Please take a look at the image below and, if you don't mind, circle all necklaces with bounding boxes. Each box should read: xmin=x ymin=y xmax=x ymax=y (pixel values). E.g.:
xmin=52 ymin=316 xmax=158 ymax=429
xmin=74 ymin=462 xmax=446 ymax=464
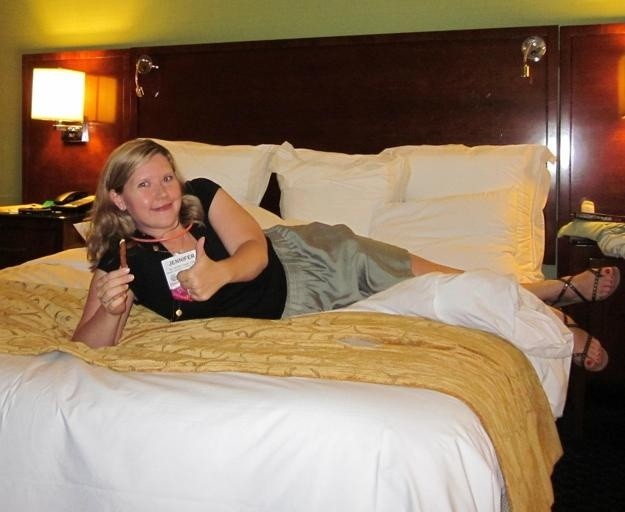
xmin=130 ymin=217 xmax=193 ymax=243
xmin=152 ymin=220 xmax=183 ymax=322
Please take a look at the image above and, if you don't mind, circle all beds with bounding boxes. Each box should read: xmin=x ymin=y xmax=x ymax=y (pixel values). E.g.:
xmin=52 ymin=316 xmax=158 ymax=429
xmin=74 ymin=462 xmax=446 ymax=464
xmin=0 ymin=217 xmax=575 ymax=510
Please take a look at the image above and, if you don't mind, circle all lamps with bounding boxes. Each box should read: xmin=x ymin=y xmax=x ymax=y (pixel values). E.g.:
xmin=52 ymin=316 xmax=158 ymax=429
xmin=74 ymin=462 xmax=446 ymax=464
xmin=520 ymin=34 xmax=548 ymax=77
xmin=133 ymin=57 xmax=160 ymax=99
xmin=30 ymin=66 xmax=90 ymax=144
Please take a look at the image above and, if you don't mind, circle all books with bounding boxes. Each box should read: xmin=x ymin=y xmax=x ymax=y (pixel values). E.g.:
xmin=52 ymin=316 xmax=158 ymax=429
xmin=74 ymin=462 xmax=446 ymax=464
xmin=573 ymin=211 xmax=625 ymax=223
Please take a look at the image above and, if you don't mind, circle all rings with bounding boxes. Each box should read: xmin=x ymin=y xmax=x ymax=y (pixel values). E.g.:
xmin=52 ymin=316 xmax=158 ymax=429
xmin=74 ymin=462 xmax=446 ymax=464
xmin=187 ymin=287 xmax=191 ymax=296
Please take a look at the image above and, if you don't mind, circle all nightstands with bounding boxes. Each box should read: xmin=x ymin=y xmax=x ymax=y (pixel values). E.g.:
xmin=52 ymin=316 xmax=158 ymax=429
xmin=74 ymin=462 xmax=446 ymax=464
xmin=1 ymin=202 xmax=90 ymax=268
xmin=556 ymin=216 xmax=625 ymax=303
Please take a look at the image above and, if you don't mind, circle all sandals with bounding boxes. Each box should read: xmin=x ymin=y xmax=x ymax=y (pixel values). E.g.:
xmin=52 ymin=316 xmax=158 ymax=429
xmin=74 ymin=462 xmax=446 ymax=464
xmin=555 ymin=307 xmax=609 ymax=372
xmin=546 ymin=267 xmax=620 ymax=306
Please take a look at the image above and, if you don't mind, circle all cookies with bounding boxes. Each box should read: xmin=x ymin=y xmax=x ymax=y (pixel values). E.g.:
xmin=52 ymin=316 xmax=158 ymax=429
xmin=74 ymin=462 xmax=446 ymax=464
xmin=119 ymin=239 xmax=127 ymax=268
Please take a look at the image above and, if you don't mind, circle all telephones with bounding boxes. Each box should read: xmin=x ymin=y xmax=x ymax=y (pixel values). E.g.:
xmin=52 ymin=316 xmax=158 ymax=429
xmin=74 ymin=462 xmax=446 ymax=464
xmin=50 ymin=190 xmax=96 ymax=211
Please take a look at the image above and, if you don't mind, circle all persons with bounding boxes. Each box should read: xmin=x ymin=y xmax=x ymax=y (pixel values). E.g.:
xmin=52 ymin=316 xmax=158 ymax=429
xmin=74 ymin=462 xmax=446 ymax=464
xmin=71 ymin=138 xmax=621 ymax=373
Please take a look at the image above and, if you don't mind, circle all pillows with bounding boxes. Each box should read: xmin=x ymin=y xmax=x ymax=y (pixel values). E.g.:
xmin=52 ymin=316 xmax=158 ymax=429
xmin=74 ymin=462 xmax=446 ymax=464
xmin=271 ymin=141 xmax=409 ymax=231
xmin=377 ymin=142 xmax=554 ymax=282
xmin=366 ymin=182 xmax=536 ymax=281
xmin=135 ymin=138 xmax=279 ymax=209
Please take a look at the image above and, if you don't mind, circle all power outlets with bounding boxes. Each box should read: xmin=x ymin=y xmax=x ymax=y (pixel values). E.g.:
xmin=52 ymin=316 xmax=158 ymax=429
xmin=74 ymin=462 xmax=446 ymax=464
xmin=581 ymin=202 xmax=594 ymax=213
